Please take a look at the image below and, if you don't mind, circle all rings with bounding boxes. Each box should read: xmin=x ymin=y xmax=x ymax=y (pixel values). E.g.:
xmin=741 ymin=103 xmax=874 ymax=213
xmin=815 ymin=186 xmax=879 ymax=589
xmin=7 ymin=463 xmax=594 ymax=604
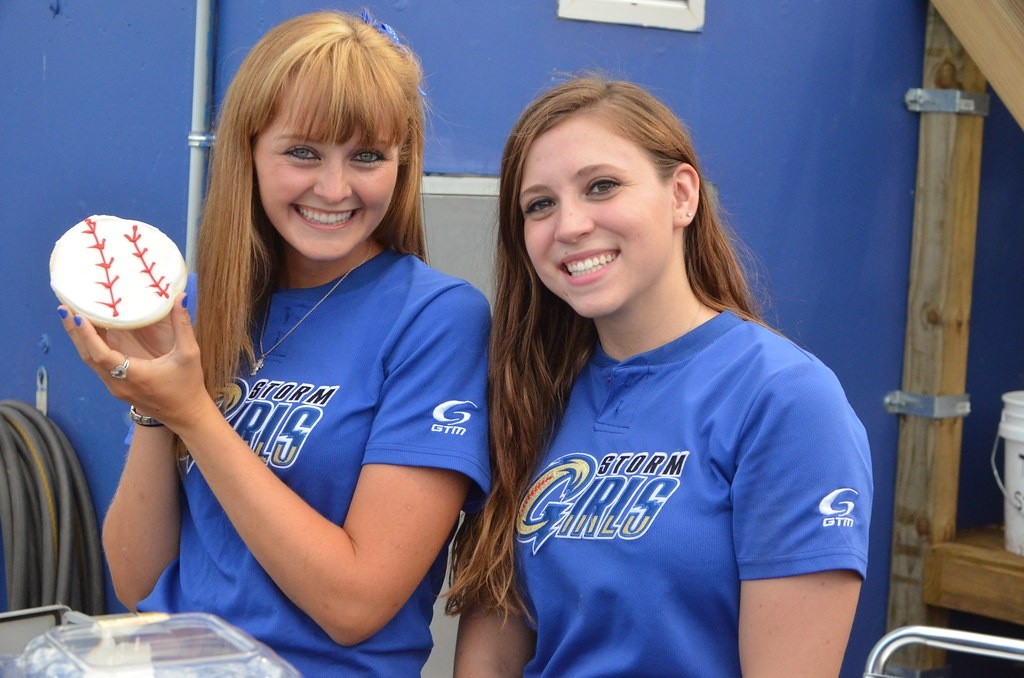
xmin=111 ymin=355 xmax=130 ymax=379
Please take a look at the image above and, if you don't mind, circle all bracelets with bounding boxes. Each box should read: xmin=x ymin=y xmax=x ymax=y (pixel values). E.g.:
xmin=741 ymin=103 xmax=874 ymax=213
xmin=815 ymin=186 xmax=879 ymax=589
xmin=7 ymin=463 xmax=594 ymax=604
xmin=130 ymin=405 xmax=163 ymax=427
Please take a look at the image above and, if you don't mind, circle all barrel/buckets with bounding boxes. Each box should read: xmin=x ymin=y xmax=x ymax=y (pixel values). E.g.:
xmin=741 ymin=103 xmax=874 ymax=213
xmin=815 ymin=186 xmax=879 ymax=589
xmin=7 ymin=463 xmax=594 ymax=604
xmin=990 ymin=390 xmax=1024 ymax=558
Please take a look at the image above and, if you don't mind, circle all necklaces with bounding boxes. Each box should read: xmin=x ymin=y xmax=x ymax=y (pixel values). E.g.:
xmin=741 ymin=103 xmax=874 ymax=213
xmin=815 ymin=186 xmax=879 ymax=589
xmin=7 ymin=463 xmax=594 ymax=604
xmin=250 ymin=241 xmax=373 ymax=376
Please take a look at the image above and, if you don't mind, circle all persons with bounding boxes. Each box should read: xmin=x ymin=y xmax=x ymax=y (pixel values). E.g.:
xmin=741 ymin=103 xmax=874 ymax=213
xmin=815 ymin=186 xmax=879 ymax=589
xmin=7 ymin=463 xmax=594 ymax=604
xmin=56 ymin=10 xmax=495 ymax=677
xmin=444 ymin=72 xmax=875 ymax=678
